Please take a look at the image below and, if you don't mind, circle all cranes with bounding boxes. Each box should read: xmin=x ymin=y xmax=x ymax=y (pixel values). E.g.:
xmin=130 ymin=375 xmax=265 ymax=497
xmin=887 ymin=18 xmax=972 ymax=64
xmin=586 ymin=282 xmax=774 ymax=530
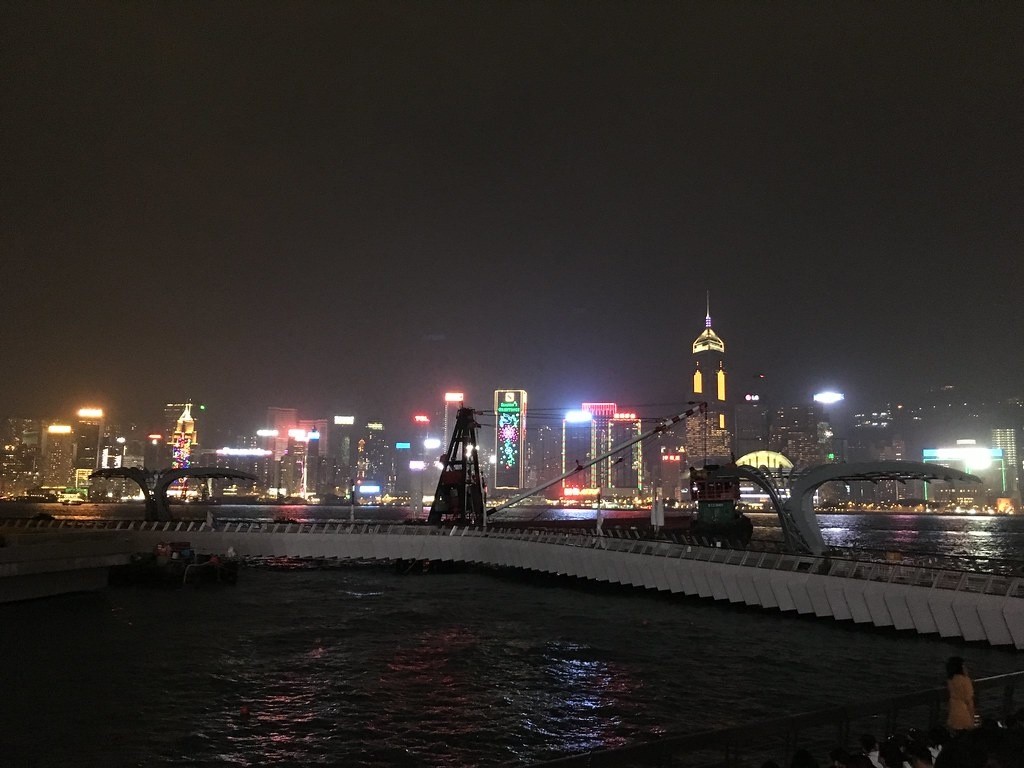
xmin=425 ymin=401 xmax=710 ymax=526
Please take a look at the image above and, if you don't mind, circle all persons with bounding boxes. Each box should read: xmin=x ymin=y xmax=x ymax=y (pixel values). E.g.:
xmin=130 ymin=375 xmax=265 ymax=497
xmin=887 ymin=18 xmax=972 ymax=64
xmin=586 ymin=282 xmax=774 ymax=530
xmin=759 ymin=681 xmax=1024 ymax=768
xmin=944 ymin=651 xmax=977 ymax=736
